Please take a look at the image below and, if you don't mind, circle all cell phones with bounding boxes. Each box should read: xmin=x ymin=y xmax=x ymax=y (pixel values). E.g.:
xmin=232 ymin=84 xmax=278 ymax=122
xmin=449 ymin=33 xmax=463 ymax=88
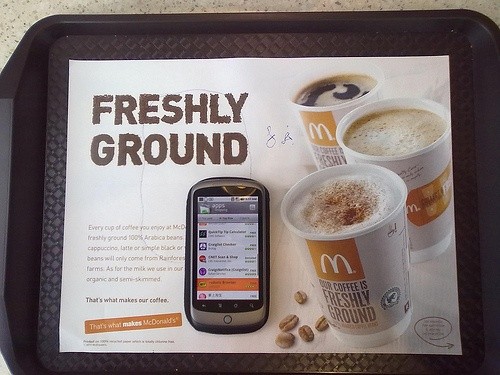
xmin=184 ymin=176 xmax=270 ymax=335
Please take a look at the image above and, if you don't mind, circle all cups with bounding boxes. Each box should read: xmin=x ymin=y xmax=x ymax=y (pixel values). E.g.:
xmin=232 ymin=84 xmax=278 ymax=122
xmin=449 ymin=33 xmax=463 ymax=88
xmin=336 ymin=95 xmax=455 ymax=262
xmin=289 ymin=74 xmax=384 ymax=171
xmin=281 ymin=163 xmax=412 ymax=348
